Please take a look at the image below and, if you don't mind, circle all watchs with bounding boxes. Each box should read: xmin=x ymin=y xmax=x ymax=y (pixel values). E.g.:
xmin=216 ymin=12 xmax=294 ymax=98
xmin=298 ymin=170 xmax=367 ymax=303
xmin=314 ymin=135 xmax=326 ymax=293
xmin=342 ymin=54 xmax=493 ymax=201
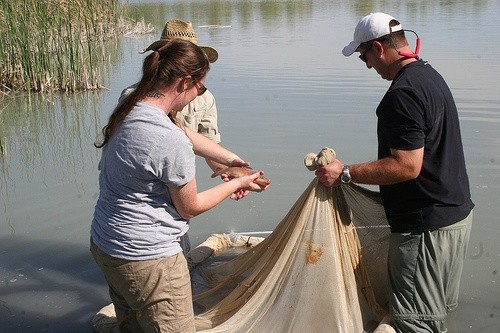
xmin=340 ymin=165 xmax=352 ymax=184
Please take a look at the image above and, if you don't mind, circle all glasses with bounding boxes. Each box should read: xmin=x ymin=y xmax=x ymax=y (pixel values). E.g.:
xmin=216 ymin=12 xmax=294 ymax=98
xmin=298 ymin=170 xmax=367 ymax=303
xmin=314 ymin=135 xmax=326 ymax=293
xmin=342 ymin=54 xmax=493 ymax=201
xmin=190 ymin=74 xmax=208 ymax=96
xmin=359 ymin=40 xmax=387 ymax=63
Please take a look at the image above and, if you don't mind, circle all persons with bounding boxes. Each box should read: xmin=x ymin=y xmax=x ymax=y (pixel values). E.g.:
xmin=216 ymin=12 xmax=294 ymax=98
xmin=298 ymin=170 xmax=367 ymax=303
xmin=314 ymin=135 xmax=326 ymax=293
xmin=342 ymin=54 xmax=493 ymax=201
xmin=89 ymin=18 xmax=269 ymax=333
xmin=315 ymin=12 xmax=475 ymax=333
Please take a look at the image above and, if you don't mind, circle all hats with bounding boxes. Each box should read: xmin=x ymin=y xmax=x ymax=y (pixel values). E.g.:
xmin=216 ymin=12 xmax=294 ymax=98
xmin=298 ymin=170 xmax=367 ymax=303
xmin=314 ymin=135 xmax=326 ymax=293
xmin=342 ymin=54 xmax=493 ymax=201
xmin=341 ymin=13 xmax=402 ymax=56
xmin=139 ymin=19 xmax=218 ymax=62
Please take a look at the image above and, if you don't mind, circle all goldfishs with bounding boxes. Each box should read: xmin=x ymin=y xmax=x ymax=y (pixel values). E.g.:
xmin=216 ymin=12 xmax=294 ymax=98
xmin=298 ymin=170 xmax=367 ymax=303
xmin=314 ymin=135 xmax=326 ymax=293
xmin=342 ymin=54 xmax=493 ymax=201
xmin=210 ymin=166 xmax=270 ymax=186
